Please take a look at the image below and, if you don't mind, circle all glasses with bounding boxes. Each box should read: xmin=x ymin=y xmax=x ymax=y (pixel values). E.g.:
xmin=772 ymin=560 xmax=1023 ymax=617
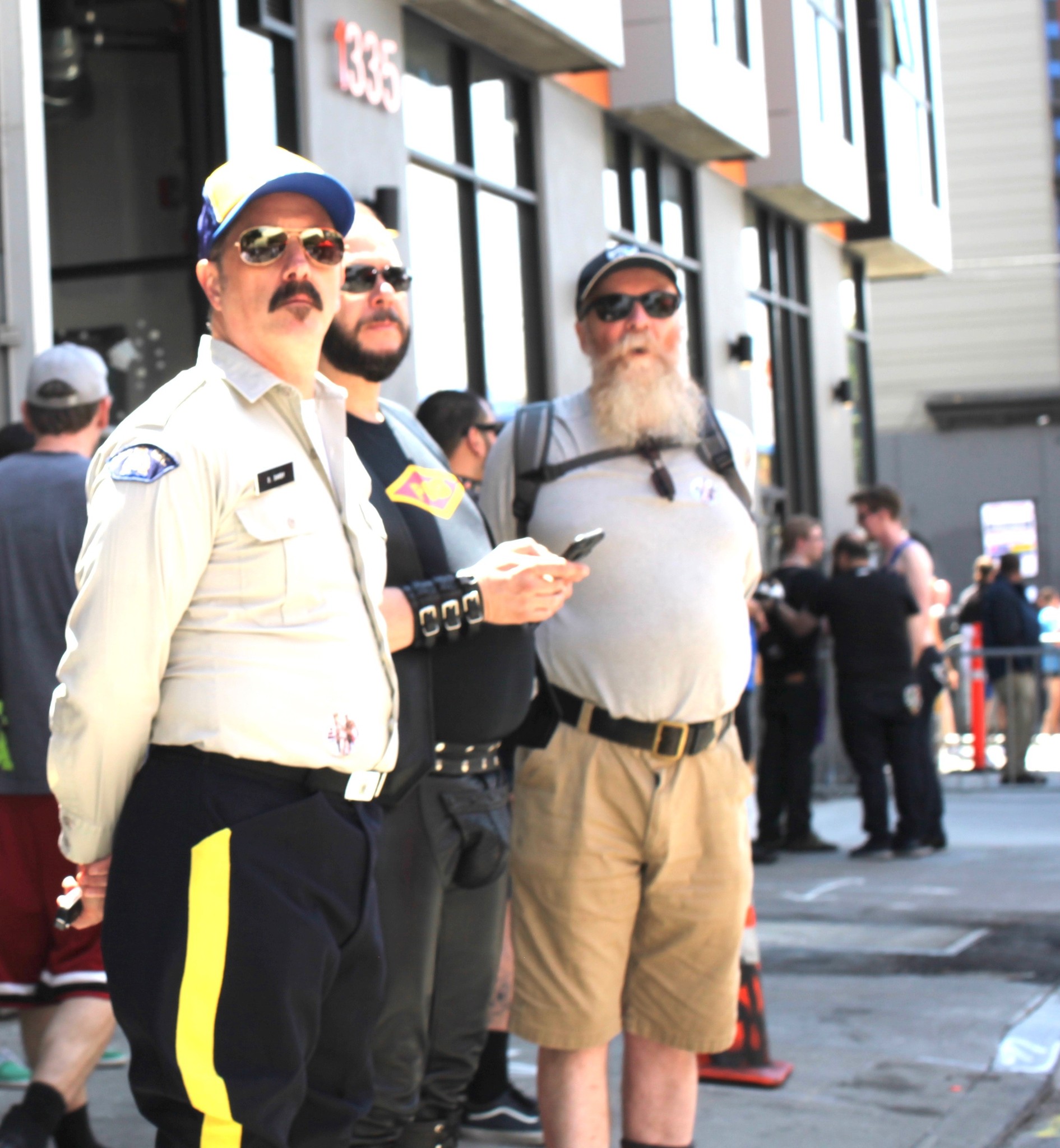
xmin=227 ymin=223 xmax=347 ymax=270
xmin=579 ymin=289 xmax=681 ymax=323
xmin=462 ymin=420 xmax=505 ymax=435
xmin=337 ymin=264 xmax=412 ymax=298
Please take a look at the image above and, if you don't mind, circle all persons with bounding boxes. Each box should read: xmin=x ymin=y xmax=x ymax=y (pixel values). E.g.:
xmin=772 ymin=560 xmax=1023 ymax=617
xmin=477 ymin=242 xmax=764 ymax=1147
xmin=0 ymin=144 xmax=1060 ymax=1148
xmin=47 ymin=139 xmax=403 ymax=1148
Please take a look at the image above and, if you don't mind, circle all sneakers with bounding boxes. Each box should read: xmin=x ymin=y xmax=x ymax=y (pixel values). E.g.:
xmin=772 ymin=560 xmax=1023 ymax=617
xmin=460 ymin=1082 xmax=546 ymax=1145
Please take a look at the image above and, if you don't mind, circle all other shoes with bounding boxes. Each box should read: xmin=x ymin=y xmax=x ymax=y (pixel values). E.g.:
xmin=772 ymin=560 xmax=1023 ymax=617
xmin=759 ymin=830 xmax=834 ymax=852
xmin=1002 ymin=773 xmax=1047 ymax=784
xmin=853 ymin=833 xmax=935 ymax=859
xmin=0 ymin=1104 xmax=49 ymax=1148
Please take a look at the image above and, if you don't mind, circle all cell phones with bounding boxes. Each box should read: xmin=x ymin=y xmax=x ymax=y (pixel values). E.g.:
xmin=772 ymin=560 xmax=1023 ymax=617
xmin=562 ymin=529 xmax=605 ymax=561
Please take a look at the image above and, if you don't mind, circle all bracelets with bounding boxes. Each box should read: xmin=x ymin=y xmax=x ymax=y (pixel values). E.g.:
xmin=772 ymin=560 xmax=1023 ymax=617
xmin=404 ymin=572 xmax=486 ymax=653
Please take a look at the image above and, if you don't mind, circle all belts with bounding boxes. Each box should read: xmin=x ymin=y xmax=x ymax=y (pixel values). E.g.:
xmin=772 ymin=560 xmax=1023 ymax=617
xmin=532 ymin=681 xmax=733 ymax=761
xmin=427 ymin=739 xmax=504 ymax=775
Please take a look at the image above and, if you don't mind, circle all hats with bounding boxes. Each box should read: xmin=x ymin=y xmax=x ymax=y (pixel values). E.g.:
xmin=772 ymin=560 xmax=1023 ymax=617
xmin=852 ymin=485 xmax=900 ymax=510
xmin=196 ymin=146 xmax=355 ymax=262
xmin=26 ymin=341 xmax=111 ymax=410
xmin=576 ymin=242 xmax=678 ymax=322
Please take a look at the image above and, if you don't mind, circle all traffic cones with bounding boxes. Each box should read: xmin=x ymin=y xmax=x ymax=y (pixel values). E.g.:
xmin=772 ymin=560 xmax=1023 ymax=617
xmin=698 ymin=904 xmax=796 ymax=1087
